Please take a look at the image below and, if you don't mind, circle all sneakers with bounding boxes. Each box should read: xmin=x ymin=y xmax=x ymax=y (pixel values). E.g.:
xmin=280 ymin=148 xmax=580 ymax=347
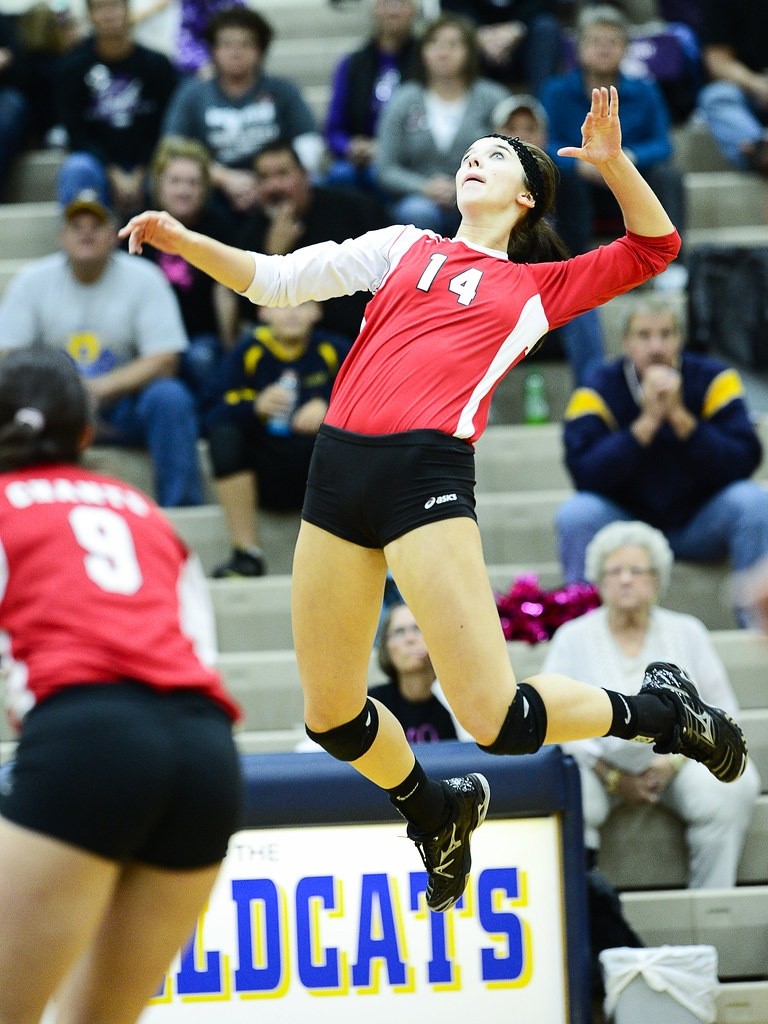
xmin=405 ymin=772 xmax=491 ymax=915
xmin=637 ymin=663 xmax=748 ymax=784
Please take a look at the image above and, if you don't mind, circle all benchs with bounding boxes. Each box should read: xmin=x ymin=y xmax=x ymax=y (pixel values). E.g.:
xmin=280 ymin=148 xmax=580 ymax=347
xmin=0 ymin=0 xmax=768 ymax=1024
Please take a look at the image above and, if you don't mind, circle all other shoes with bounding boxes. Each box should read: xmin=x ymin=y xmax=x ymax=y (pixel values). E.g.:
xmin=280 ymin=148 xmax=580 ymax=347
xmin=208 ymin=546 xmax=264 ymax=580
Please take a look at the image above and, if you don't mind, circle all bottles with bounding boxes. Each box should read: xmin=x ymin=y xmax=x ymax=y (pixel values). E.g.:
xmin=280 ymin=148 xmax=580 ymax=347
xmin=523 ymin=364 xmax=551 ymax=424
xmin=267 ymin=368 xmax=299 ymax=438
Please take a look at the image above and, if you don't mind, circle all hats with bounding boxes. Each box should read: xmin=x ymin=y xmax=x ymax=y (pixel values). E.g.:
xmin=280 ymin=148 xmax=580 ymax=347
xmin=63 ymin=184 xmax=123 ymax=230
xmin=493 ymin=93 xmax=550 ymax=133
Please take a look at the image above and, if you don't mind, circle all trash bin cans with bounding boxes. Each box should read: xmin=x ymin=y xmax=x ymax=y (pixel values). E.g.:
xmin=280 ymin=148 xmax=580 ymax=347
xmin=601 ymin=943 xmax=717 ymax=1024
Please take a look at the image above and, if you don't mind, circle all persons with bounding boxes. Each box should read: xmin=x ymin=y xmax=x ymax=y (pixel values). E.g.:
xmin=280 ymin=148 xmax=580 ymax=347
xmin=362 ymin=604 xmax=473 ymax=744
xmin=550 ymin=297 xmax=768 ymax=631
xmin=118 ymin=85 xmax=749 ymax=915
xmin=0 ymin=344 xmax=251 ymax=1024
xmin=532 ymin=519 xmax=763 ymax=891
xmin=0 ymin=0 xmax=345 ymax=577
xmin=324 ymin=0 xmax=768 ymax=393
